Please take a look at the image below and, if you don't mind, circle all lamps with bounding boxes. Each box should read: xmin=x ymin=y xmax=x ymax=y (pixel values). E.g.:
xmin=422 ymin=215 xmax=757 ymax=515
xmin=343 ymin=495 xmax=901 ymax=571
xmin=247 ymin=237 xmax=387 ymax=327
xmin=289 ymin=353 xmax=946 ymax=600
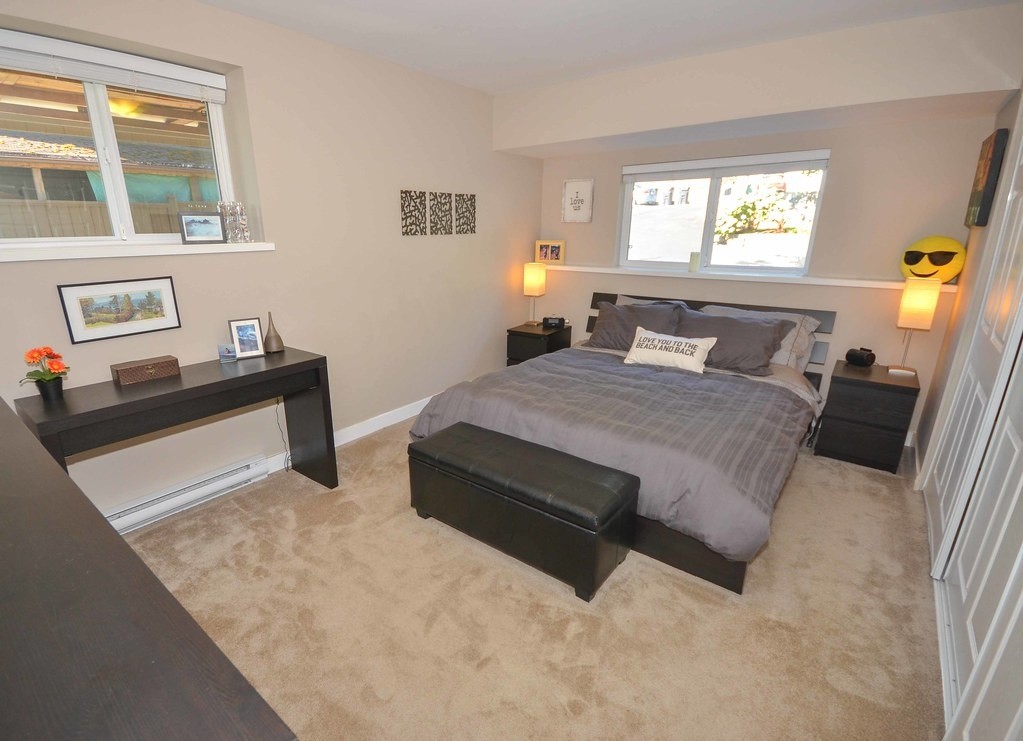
xmin=888 ymin=278 xmax=943 ymax=375
xmin=523 ymin=263 xmax=545 ymax=326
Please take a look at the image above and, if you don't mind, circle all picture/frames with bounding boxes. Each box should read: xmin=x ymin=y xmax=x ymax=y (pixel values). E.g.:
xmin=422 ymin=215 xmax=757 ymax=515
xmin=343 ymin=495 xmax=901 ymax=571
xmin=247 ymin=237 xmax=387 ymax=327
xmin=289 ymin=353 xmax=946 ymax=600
xmin=536 ymin=239 xmax=565 ymax=264
xmin=56 ymin=276 xmax=182 ymax=345
xmin=180 ymin=214 xmax=226 ymax=244
xmin=562 ymin=177 xmax=595 ymax=222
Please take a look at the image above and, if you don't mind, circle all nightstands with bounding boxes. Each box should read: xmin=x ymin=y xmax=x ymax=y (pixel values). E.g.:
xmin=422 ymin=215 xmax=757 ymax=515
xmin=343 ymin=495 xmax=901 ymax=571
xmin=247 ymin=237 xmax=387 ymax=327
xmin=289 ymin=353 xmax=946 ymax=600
xmin=507 ymin=321 xmax=571 ymax=367
xmin=813 ymin=360 xmax=920 ymax=474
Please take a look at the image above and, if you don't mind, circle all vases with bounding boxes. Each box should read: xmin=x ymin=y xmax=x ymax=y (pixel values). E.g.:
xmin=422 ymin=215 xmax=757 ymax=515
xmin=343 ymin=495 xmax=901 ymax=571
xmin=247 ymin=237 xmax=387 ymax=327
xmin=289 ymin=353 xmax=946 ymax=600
xmin=34 ymin=377 xmax=64 ymax=400
xmin=264 ymin=311 xmax=284 ymax=352
xmin=228 ymin=318 xmax=266 ymax=360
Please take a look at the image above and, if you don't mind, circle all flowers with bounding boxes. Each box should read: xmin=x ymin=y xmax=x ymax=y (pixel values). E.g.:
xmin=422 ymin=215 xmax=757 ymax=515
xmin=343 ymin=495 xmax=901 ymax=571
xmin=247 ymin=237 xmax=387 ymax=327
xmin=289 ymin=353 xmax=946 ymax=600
xmin=19 ymin=346 xmax=70 ymax=383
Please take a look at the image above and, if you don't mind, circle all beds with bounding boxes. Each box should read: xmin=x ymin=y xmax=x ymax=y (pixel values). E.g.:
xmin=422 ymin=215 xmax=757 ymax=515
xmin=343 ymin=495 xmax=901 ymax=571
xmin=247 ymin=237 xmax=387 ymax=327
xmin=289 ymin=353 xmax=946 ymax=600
xmin=408 ymin=289 xmax=837 ymax=597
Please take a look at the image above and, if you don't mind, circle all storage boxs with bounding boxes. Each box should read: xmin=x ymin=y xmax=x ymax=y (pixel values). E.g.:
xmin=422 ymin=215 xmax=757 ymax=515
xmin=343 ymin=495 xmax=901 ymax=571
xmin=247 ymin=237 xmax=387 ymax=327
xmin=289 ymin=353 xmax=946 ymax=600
xmin=110 ymin=355 xmax=181 ymax=386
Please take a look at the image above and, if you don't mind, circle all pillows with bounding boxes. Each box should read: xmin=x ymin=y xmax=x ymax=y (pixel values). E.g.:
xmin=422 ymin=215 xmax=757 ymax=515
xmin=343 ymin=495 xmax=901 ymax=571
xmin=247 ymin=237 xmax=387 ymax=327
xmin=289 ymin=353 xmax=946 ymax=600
xmin=617 ymin=293 xmax=686 ymax=305
xmin=624 ymin=325 xmax=717 ymax=373
xmin=580 ymin=301 xmax=675 ymax=350
xmin=674 ymin=304 xmax=797 ymax=375
xmin=699 ymin=304 xmax=820 ymax=369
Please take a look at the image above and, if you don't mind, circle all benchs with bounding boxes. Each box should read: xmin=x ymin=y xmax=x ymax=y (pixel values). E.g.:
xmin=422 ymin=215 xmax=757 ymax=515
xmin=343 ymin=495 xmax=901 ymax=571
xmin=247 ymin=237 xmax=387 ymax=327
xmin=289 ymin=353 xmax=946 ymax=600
xmin=408 ymin=420 xmax=640 ymax=603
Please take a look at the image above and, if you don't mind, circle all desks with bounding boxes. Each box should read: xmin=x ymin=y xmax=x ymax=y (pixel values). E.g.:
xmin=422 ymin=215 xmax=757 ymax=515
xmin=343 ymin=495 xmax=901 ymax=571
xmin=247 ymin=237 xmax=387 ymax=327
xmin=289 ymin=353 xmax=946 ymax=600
xmin=14 ymin=345 xmax=339 ymax=490
